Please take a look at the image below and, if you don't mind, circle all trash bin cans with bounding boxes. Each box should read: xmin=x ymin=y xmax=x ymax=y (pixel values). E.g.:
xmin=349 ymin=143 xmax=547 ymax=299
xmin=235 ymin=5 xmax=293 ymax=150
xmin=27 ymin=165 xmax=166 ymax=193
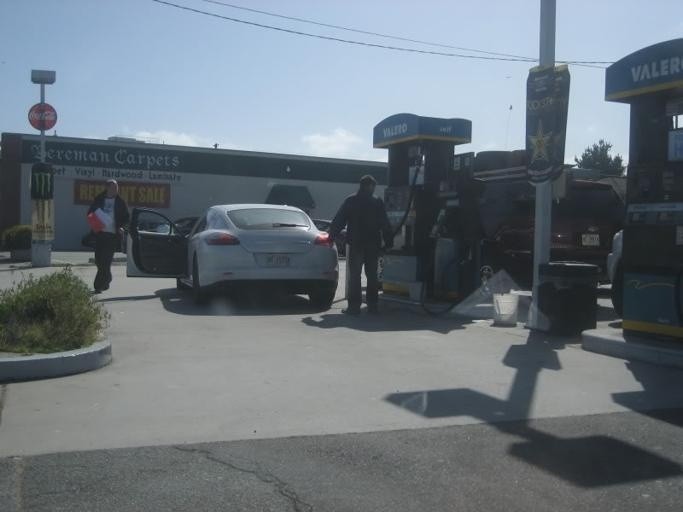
xmin=537 ymin=260 xmax=599 ymax=337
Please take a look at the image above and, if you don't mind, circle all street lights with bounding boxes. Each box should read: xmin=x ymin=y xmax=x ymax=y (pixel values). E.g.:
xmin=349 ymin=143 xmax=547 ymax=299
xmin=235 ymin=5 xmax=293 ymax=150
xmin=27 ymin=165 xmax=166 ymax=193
xmin=28 ymin=68 xmax=57 ymax=270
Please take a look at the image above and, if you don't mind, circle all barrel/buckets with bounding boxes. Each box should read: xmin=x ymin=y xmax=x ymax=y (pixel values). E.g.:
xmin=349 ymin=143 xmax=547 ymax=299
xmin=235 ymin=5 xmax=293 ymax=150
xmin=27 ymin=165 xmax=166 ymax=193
xmin=493 ymin=293 xmax=520 ymax=327
xmin=408 ymin=281 xmax=423 ymax=302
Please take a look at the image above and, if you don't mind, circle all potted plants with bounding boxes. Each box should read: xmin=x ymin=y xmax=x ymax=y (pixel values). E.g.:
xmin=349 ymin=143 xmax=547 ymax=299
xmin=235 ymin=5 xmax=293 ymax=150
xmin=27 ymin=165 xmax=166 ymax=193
xmin=3 ymin=223 xmax=31 ymax=262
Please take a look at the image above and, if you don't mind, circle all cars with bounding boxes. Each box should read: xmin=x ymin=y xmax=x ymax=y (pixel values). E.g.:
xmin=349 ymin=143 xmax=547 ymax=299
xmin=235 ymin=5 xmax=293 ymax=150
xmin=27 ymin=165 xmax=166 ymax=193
xmin=79 ymin=215 xmax=203 ymax=256
xmin=309 ymin=216 xmax=349 ymax=256
xmin=124 ymin=203 xmax=338 ymax=310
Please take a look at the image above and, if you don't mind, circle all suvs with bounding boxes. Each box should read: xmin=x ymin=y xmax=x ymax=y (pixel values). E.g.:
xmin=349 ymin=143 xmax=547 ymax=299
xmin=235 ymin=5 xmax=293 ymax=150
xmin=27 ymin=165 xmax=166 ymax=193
xmin=362 ymin=165 xmax=627 ymax=304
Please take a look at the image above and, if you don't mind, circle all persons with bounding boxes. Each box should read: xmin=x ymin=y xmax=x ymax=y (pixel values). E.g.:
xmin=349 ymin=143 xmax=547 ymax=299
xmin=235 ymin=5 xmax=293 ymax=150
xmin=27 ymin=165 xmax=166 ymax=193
xmin=327 ymin=176 xmax=392 ymax=315
xmin=87 ymin=179 xmax=130 ymax=293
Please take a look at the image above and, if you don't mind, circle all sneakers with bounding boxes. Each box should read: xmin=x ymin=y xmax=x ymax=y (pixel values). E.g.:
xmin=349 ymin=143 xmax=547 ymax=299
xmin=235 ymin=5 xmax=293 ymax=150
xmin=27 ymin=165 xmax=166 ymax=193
xmin=94 ymin=278 xmax=112 ymax=294
xmin=342 ymin=305 xmax=377 ymax=315
